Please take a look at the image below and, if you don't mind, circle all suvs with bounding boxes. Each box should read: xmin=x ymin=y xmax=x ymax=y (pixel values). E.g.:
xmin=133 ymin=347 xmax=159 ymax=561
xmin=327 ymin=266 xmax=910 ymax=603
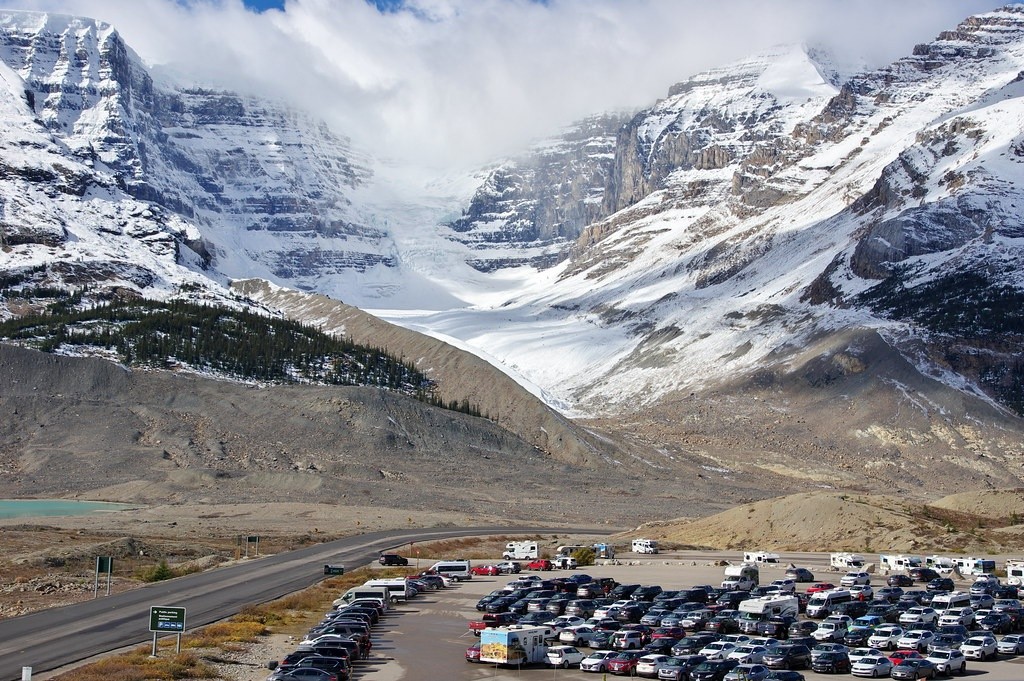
xmin=265 ymin=559 xmax=473 ymax=681
xmin=379 ymin=555 xmax=409 ymax=566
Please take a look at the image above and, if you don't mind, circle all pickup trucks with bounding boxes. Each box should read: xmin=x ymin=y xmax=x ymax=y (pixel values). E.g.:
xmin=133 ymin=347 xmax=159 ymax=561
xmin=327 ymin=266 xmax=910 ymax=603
xmin=468 ymin=613 xmax=517 ymax=637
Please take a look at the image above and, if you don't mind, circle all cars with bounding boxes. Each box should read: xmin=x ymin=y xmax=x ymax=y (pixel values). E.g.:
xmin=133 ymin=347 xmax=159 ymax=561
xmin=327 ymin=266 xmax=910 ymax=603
xmin=476 ymin=550 xmax=1024 ymax=681
xmin=465 ymin=640 xmax=482 ymax=662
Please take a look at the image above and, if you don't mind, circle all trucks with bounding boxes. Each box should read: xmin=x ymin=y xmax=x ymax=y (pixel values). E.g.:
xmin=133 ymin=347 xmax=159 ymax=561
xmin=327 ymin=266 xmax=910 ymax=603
xmin=555 ymin=544 xmax=593 ymax=559
xmin=502 ymin=539 xmax=540 ymax=560
xmin=632 ymin=539 xmax=659 ymax=554
xmin=594 ymin=543 xmax=616 ymax=559
